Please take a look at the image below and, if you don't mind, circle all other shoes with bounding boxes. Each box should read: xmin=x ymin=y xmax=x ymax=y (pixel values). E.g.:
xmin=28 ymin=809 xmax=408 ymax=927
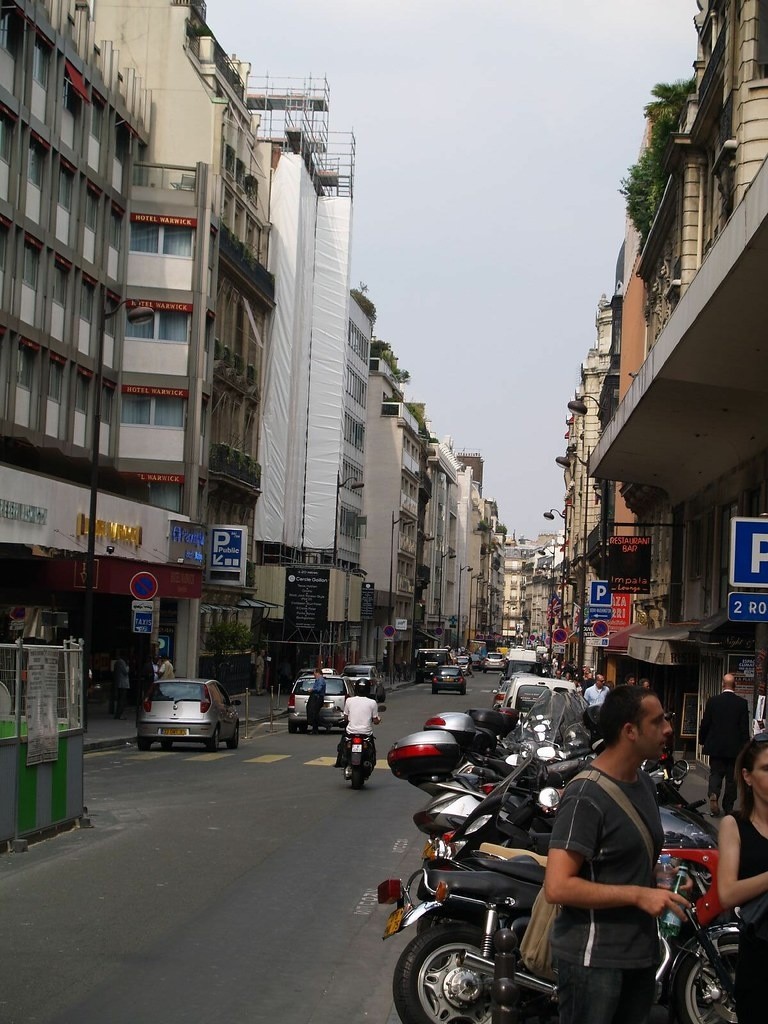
xmin=309 ymin=729 xmax=317 ymax=734
xmin=326 ymin=722 xmax=333 ymax=734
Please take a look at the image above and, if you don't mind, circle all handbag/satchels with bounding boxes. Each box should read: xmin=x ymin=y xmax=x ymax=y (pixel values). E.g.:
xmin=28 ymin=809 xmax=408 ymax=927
xmin=519 ymin=881 xmax=563 ymax=978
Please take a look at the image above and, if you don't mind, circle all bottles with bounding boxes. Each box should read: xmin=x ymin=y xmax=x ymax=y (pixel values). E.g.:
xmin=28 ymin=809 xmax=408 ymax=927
xmin=660 ymin=862 xmax=688 ymax=936
xmin=657 ymin=855 xmax=674 ymax=890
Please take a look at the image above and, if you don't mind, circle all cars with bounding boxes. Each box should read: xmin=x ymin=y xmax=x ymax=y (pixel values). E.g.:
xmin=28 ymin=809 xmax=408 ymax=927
xmin=481 ymin=651 xmax=508 ymax=674
xmin=430 ymin=664 xmax=469 ymax=695
xmin=134 ymin=678 xmax=242 ymax=752
xmin=287 ymin=673 xmax=356 ymax=734
xmin=341 ymin=661 xmax=386 ymax=703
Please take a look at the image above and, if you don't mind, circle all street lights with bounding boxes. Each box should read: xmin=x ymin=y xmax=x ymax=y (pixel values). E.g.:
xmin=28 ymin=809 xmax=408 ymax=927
xmin=384 ymin=510 xmax=413 ymax=676
xmin=537 ymin=544 xmax=558 ymax=677
xmin=76 ymin=286 xmax=154 ymax=735
xmin=467 ymin=571 xmax=502 ymax=642
xmin=436 ymin=540 xmax=458 ymax=649
xmin=455 ymin=563 xmax=474 ymax=651
xmin=567 ymin=383 xmax=612 ymax=682
xmin=555 ymin=443 xmax=591 ymax=684
xmin=543 ymin=503 xmax=568 ymax=676
xmin=332 ymin=469 xmax=365 ymax=566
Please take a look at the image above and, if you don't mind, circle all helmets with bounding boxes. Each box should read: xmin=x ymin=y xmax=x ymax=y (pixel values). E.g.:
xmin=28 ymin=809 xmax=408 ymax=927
xmin=354 ymin=679 xmax=371 ymax=696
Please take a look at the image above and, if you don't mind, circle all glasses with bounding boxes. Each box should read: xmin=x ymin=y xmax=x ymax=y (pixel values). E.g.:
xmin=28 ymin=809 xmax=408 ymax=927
xmin=597 ymin=679 xmax=604 ymax=683
xmin=752 ymin=733 xmax=768 ymax=741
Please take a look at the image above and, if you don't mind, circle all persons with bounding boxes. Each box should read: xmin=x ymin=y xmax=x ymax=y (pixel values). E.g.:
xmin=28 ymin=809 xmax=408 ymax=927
xmin=698 ymin=673 xmax=749 ymax=816
xmin=639 ymin=679 xmax=650 ymax=688
xmin=396 ymin=657 xmax=407 ymax=680
xmin=144 ymin=654 xmax=174 ymax=681
xmin=585 ymin=674 xmax=609 ymax=707
xmin=308 ymin=668 xmax=333 ymax=735
xmin=624 ymin=673 xmax=636 ymax=685
xmin=255 ymin=650 xmax=266 ymax=695
xmin=114 ymin=651 xmax=130 ymax=720
xmin=555 ymin=658 xmax=596 ymax=696
xmin=717 ymin=732 xmax=768 ymax=1024
xmin=544 ymin=685 xmax=694 ymax=1024
xmin=278 ymin=656 xmax=292 ymax=692
xmin=335 ymin=678 xmax=381 ymax=767
xmin=466 ymin=653 xmax=475 ymax=678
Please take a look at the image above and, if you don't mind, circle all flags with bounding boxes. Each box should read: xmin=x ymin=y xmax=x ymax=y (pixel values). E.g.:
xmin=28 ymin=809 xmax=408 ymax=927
xmin=547 ymin=593 xmax=561 ymax=625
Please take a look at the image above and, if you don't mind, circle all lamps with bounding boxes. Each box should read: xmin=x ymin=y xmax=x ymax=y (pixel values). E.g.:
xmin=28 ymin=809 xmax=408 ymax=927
xmin=106 ymin=545 xmax=115 ymax=555
xmin=177 ymin=557 xmax=184 ymax=563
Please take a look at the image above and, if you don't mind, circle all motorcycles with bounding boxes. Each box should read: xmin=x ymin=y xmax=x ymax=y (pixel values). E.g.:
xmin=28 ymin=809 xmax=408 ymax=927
xmin=332 ymin=705 xmax=387 ymax=789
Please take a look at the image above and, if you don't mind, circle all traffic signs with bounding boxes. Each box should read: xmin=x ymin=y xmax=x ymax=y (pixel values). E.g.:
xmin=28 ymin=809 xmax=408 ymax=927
xmin=588 ymin=579 xmax=612 ymax=620
xmin=204 ymin=523 xmax=249 ymax=586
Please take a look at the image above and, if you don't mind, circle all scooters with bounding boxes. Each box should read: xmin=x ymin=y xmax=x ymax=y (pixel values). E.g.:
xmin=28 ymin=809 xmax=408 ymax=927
xmin=375 ymin=643 xmax=745 ymax=1024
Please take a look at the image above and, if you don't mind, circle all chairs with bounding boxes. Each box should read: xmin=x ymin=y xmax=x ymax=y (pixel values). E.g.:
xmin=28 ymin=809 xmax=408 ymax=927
xmin=170 ymin=173 xmax=196 ymax=190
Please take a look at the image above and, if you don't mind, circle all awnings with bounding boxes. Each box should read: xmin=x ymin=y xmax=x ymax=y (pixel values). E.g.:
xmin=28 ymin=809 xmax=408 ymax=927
xmin=627 ymin=625 xmax=697 ymax=665
xmin=604 ymin=622 xmax=642 ymax=656
xmin=688 ymin=607 xmax=755 ymax=651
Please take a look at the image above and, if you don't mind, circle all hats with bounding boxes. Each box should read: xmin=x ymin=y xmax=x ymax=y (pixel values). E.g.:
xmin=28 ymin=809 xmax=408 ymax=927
xmin=158 ymin=652 xmax=170 ymax=659
